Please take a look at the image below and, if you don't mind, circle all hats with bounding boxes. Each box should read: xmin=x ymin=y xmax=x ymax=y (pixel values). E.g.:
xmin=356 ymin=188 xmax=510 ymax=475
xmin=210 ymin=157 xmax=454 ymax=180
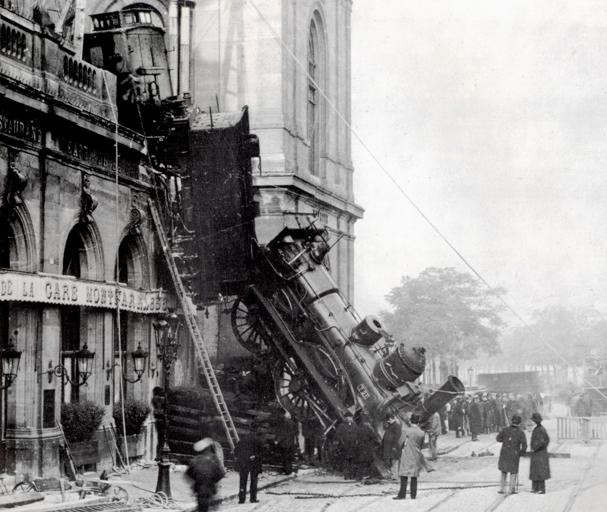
xmin=511 ymin=415 xmax=521 ymax=423
xmin=411 ymin=414 xmax=419 ymax=423
xmin=385 ymin=413 xmax=393 ymax=419
xmin=530 ymin=414 xmax=542 ymax=421
xmin=344 ymin=412 xmax=354 ymax=417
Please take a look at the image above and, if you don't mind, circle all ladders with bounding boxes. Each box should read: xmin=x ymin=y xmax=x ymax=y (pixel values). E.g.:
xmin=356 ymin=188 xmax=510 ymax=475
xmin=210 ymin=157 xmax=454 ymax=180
xmin=146 ymin=198 xmax=240 ymax=454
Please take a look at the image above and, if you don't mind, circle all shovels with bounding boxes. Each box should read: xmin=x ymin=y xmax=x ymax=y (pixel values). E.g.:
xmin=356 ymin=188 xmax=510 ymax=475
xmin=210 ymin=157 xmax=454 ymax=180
xmin=103 ymin=424 xmax=118 ymax=473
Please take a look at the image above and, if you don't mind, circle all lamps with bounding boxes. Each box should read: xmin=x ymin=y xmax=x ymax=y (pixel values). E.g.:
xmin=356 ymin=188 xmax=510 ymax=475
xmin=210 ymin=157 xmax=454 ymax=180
xmin=0 ymin=338 xmax=22 ymax=390
xmin=48 ymin=342 xmax=96 ymax=386
xmin=106 ymin=341 xmax=148 ymax=383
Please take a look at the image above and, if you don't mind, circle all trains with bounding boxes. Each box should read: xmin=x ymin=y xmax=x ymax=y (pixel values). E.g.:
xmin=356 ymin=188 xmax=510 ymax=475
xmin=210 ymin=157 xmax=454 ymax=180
xmin=82 ymin=7 xmax=463 ymax=484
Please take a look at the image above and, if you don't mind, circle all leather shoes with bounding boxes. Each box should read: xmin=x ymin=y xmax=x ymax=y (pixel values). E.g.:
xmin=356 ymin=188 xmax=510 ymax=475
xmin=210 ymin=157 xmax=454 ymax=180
xmin=392 ymin=495 xmax=415 ymax=499
xmin=498 ymin=490 xmax=545 ymax=494
xmin=238 ymin=496 xmax=260 ymax=504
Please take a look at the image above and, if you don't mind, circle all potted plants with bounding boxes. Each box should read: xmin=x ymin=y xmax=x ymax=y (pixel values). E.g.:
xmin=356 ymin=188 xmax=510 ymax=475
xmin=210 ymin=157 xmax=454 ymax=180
xmin=113 ymin=399 xmax=151 ymax=458
xmin=60 ymin=400 xmax=105 ymax=466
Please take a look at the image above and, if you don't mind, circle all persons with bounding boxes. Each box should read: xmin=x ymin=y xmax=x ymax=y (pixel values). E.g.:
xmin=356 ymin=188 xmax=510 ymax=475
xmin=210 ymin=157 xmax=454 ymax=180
xmin=184 ymin=446 xmax=225 ymax=512
xmin=332 ymin=412 xmax=360 ymax=480
xmin=424 ymin=389 xmax=544 ymax=441
xmin=391 ymin=414 xmax=436 ymax=500
xmin=232 ymin=428 xmax=265 ymax=504
xmin=382 ymin=412 xmax=403 ymax=479
xmin=357 ymin=413 xmax=382 ymax=479
xmin=529 ymin=412 xmax=552 ymax=494
xmin=495 ymin=414 xmax=528 ymax=494
